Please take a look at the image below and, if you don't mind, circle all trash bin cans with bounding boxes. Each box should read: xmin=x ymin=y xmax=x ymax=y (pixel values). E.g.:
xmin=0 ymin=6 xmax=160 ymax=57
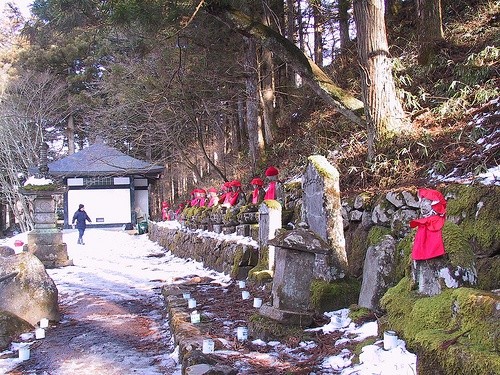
xmin=138 ymin=221 xmax=147 ymax=234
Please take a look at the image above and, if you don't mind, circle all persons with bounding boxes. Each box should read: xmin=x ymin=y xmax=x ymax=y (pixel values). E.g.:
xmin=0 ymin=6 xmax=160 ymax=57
xmin=161 ymin=165 xmax=446 ymax=263
xmin=72 ymin=203 xmax=92 ymax=245
